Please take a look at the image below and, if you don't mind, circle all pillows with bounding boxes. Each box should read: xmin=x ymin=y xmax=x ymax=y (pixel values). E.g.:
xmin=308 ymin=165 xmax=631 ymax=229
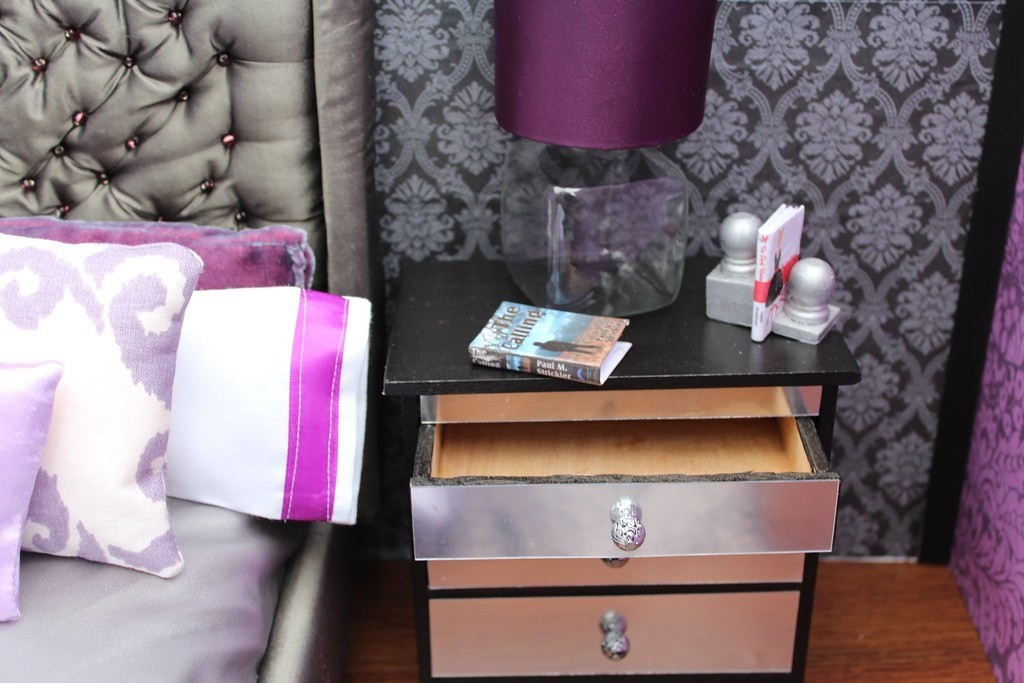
xmin=1 ymin=215 xmax=317 ymax=292
xmin=0 ymin=234 xmax=205 ymax=579
xmin=163 ymin=283 xmax=372 ymax=524
xmin=0 ymin=356 xmax=66 ymax=622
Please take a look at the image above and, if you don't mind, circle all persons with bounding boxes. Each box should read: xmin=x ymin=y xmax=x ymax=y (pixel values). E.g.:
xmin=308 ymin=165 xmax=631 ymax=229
xmin=766 ymin=226 xmax=784 ymax=307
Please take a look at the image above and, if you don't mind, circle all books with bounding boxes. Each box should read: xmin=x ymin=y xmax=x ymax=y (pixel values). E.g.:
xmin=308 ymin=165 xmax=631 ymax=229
xmin=468 ymin=301 xmax=632 ymax=386
xmin=751 ymin=204 xmax=805 ymax=342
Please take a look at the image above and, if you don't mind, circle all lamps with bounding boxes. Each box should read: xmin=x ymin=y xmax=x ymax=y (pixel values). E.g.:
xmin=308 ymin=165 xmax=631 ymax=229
xmin=491 ymin=0 xmax=720 ymax=318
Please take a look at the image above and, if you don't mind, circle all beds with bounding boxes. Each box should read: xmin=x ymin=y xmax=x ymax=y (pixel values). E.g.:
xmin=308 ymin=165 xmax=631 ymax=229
xmin=1 ymin=2 xmax=374 ymax=682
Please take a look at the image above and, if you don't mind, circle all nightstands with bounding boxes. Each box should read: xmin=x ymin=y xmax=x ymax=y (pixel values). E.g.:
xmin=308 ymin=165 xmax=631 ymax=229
xmin=380 ymin=261 xmax=862 ymax=683
